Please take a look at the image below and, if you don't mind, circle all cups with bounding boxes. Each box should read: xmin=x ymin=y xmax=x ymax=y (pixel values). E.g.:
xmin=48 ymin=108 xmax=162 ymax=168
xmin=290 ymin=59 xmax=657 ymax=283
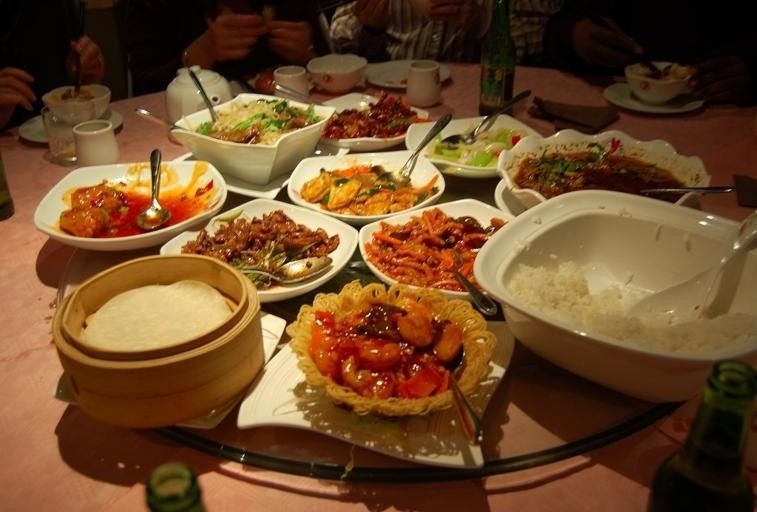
xmin=272 ymin=63 xmax=308 ymax=101
xmin=403 ymin=59 xmax=444 ymax=108
xmin=70 ymin=118 xmax=119 ymax=168
xmin=39 ymin=97 xmax=97 ymax=160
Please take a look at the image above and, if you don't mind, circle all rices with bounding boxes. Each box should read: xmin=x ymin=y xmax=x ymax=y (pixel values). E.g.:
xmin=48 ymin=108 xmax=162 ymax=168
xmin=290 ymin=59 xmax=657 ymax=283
xmin=506 ymin=259 xmax=756 ymax=354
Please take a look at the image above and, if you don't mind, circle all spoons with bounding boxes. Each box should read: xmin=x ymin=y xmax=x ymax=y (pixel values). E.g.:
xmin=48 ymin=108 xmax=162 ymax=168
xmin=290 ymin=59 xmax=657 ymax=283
xmin=622 ymin=208 xmax=757 ymax=325
xmin=372 ymin=110 xmax=453 ymax=192
xmin=435 ymin=89 xmax=534 ymax=150
xmin=238 ymin=253 xmax=334 ymax=287
xmin=135 ymin=147 xmax=173 ymax=230
xmin=441 ymin=347 xmax=489 ymax=448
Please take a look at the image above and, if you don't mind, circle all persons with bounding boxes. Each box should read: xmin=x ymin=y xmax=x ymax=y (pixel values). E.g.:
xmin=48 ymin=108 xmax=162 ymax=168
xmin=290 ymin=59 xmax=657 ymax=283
xmin=319 ymin=0 xmax=565 ymax=68
xmin=1 ymin=2 xmax=130 ymax=131
xmin=115 ymin=0 xmax=333 ymax=104
xmin=539 ymin=0 xmax=755 ymax=105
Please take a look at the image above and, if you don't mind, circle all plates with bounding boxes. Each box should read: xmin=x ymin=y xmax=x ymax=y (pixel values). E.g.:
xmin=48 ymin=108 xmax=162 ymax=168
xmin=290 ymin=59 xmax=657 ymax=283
xmin=34 ymin=161 xmax=226 ymax=249
xmin=289 ymin=151 xmax=447 ymax=222
xmin=239 ymin=315 xmax=516 ymax=469
xmin=497 ymin=129 xmax=713 ymax=212
xmin=608 ymin=81 xmax=704 ymax=115
xmin=409 ymin=115 xmax=543 ymax=178
xmin=19 ymin=107 xmax=125 ymax=143
xmin=494 ymin=179 xmax=527 ymax=218
xmin=52 ymin=305 xmax=288 ymax=430
xmin=160 ymin=199 xmax=359 ymax=300
xmin=166 ymin=145 xmax=352 ymax=200
xmin=362 ymin=199 xmax=514 ymax=298
xmin=368 ymin=58 xmax=453 ymax=89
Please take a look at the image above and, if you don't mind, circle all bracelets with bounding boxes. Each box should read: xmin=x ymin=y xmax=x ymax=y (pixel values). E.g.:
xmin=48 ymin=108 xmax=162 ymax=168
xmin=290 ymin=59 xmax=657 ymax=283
xmin=182 ymin=47 xmax=192 ymax=68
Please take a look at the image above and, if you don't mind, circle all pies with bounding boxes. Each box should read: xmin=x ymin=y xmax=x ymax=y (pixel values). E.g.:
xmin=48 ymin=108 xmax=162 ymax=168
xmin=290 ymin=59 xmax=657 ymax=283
xmin=82 ymin=280 xmax=234 ymax=351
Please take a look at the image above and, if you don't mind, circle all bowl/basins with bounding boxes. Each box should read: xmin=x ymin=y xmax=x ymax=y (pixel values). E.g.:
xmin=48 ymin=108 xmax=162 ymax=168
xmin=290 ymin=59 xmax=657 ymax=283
xmin=172 ymin=93 xmax=337 ymax=182
xmin=42 ymin=83 xmax=110 ymax=122
xmin=625 ymin=60 xmax=696 ymax=104
xmin=470 ymin=189 xmax=755 ymax=399
xmin=306 ymin=52 xmax=368 ymax=96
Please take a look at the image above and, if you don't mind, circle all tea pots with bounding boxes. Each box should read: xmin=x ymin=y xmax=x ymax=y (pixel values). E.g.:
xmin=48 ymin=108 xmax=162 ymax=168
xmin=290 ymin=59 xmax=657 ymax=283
xmin=164 ymin=66 xmax=232 ymax=123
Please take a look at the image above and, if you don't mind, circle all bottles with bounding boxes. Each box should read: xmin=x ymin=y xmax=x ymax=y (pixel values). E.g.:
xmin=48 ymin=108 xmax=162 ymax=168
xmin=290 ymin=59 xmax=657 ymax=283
xmin=476 ymin=0 xmax=517 ymax=118
xmin=140 ymin=459 xmax=207 ymax=511
xmin=645 ymin=356 xmax=755 ymax=512
